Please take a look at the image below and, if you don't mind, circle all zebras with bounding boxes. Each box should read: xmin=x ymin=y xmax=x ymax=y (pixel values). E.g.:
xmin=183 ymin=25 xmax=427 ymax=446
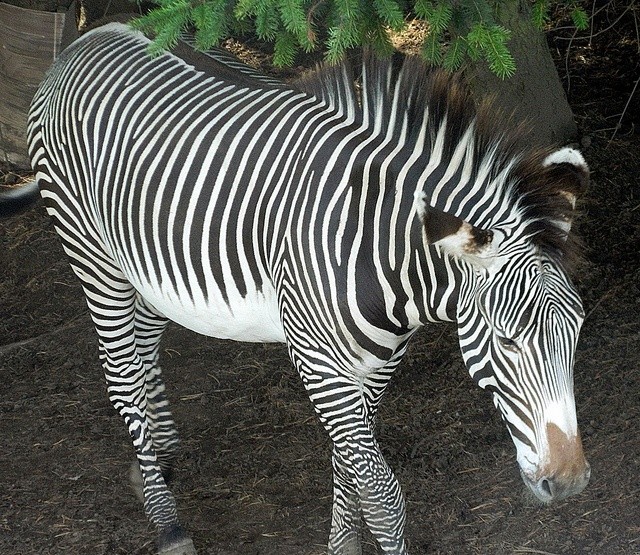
xmin=25 ymin=14 xmax=591 ymax=555
